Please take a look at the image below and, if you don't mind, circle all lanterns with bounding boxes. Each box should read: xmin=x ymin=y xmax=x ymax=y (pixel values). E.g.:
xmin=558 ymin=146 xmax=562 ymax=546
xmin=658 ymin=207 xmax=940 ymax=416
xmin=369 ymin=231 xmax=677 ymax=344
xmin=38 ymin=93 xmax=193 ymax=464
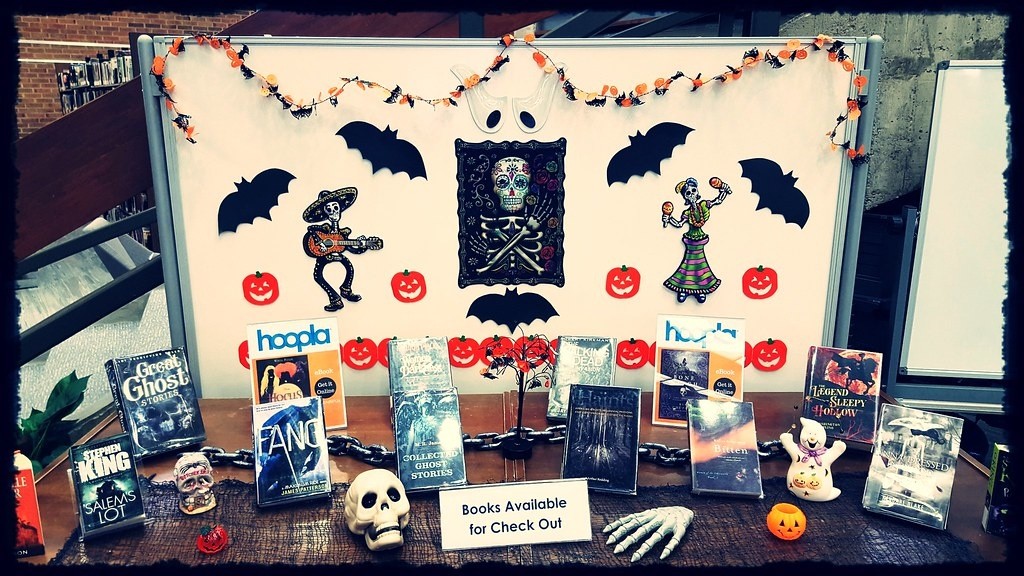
xmin=767 ymin=503 xmax=806 ymax=541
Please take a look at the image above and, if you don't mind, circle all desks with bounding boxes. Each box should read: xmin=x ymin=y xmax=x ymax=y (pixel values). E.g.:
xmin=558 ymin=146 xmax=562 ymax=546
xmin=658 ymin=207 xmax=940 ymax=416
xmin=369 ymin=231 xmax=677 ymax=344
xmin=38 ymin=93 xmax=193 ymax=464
xmin=17 ymin=390 xmax=1010 ymax=566
